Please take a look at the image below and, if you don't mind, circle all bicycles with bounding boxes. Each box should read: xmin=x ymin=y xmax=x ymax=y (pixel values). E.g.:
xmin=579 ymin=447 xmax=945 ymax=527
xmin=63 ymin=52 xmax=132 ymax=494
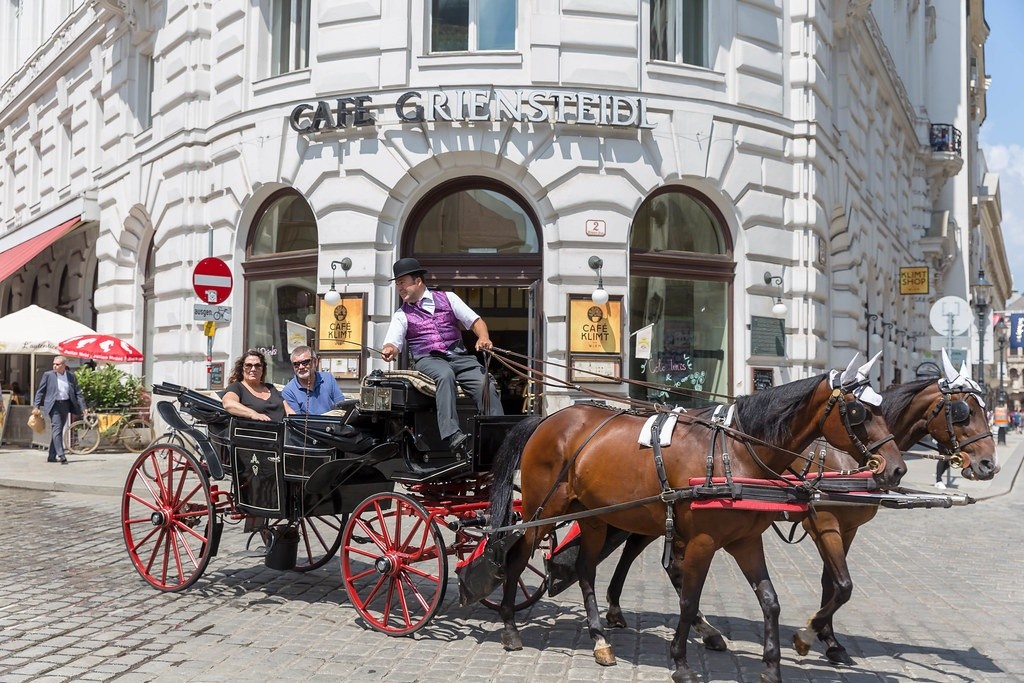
xmin=64 ymin=400 xmax=156 ymax=454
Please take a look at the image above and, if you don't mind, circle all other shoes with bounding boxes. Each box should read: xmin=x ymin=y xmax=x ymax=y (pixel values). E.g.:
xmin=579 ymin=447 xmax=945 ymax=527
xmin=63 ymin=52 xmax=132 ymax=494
xmin=60 ymin=457 xmax=66 ymax=464
xmin=935 ymin=481 xmax=947 ymax=490
xmin=448 ymin=433 xmax=471 ymax=449
xmin=48 ymin=459 xmax=59 ymax=462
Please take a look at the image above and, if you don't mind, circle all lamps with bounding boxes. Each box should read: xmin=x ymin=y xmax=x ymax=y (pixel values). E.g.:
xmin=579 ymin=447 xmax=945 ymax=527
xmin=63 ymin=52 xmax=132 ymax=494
xmin=305 ymin=305 xmax=316 ymax=328
xmin=588 ymin=255 xmax=610 ymax=307
xmin=764 ymin=271 xmax=787 ymax=317
xmin=325 ymin=257 xmax=352 ymax=306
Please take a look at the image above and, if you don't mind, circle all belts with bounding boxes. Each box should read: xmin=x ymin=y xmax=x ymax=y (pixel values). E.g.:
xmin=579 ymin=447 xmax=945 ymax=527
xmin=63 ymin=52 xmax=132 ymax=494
xmin=54 ymin=400 xmax=68 ymax=403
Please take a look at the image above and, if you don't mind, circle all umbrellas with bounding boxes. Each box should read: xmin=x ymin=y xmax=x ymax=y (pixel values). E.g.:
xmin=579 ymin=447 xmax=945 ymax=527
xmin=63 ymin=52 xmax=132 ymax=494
xmin=58 ymin=335 xmax=144 ymax=367
xmin=0 ymin=304 xmax=97 ymax=406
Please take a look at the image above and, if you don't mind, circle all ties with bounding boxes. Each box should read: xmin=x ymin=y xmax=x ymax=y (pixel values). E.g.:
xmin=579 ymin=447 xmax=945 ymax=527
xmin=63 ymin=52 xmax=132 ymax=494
xmin=414 ymin=301 xmax=432 ymax=316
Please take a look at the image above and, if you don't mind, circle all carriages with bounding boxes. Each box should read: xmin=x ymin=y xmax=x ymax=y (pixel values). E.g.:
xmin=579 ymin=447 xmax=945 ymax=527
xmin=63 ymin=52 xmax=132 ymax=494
xmin=121 ymin=348 xmax=1001 ymax=683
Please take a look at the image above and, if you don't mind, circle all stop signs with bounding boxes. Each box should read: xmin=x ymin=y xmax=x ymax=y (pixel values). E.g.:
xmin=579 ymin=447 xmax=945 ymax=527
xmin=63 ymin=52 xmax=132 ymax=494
xmin=192 ymin=257 xmax=233 ymax=305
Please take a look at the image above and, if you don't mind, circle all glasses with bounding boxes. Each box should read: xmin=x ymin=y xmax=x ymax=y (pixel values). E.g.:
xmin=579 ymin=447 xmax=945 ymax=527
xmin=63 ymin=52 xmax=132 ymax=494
xmin=243 ymin=363 xmax=263 ymax=368
xmin=52 ymin=363 xmax=60 ymax=365
xmin=292 ymin=358 xmax=313 ymax=368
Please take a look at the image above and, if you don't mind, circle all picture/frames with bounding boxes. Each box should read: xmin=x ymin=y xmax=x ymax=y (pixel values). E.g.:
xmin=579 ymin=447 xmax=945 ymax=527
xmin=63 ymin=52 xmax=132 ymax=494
xmin=659 ymin=315 xmax=695 ymax=376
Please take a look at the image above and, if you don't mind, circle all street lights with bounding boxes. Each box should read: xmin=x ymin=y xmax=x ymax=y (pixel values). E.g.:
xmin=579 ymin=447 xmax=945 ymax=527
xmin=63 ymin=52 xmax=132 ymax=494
xmin=994 ymin=317 xmax=1009 ymax=402
xmin=968 ymin=265 xmax=994 ymax=401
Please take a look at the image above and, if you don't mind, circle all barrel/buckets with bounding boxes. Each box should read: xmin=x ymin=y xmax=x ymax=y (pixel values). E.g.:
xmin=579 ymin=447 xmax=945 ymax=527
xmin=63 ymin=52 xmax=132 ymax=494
xmin=266 ymin=525 xmax=300 ymax=569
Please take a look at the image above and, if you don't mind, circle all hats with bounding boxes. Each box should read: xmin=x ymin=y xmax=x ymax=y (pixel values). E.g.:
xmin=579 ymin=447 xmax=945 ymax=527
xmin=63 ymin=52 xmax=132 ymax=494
xmin=388 ymin=258 xmax=427 ymax=281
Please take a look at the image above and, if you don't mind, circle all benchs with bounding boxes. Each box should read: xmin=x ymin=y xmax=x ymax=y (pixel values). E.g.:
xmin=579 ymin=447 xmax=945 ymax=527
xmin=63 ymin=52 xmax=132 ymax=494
xmin=370 ymin=368 xmax=479 ymax=461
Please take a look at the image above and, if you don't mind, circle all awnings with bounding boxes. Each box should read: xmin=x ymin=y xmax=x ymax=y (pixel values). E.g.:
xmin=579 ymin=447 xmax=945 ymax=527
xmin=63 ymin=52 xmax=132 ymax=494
xmin=0 ymin=215 xmax=80 ymax=284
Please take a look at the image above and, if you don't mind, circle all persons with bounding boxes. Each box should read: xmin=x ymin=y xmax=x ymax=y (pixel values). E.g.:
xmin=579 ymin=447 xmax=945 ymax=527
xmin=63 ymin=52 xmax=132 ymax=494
xmin=281 ymin=346 xmax=345 ymax=415
xmin=935 ymin=129 xmax=951 ymax=151
xmin=2 ymin=382 xmax=21 ymax=405
xmin=382 ymin=258 xmax=503 ymax=456
xmin=31 ymin=357 xmax=88 ymax=464
xmin=931 ymin=438 xmax=953 ymax=490
xmin=221 ymin=350 xmax=285 ymax=422
xmin=987 ymin=408 xmax=1024 ymax=434
